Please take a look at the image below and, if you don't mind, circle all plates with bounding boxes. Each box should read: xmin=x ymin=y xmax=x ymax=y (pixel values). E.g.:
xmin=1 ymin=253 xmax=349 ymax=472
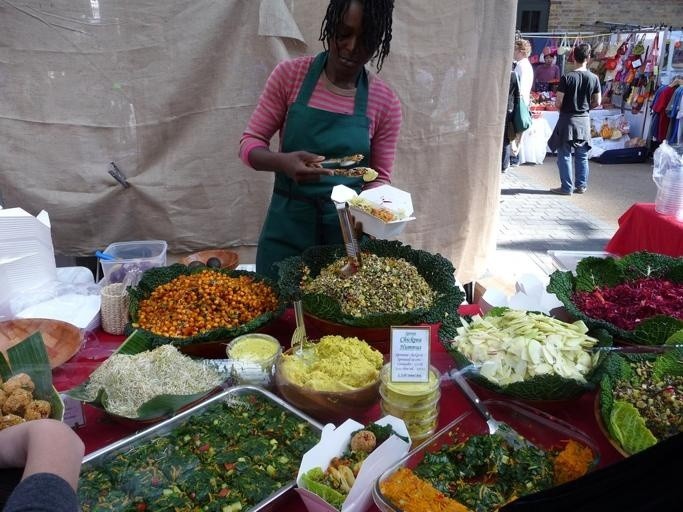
xmin=0 ymin=317 xmax=84 ymax=374
xmin=590 ymin=390 xmax=633 ymax=458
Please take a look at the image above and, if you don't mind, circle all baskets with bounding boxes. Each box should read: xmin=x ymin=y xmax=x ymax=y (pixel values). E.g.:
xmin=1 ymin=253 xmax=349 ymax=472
xmin=101 ymin=283 xmax=130 ymax=335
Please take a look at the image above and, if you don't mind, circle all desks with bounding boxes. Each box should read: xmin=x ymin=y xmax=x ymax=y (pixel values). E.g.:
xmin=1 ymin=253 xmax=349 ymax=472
xmin=613 ymin=201 xmax=683 ymax=260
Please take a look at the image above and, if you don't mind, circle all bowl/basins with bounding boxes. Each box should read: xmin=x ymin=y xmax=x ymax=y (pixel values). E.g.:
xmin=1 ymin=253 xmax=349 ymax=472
xmin=654 ymin=169 xmax=682 ymax=216
xmin=301 ymin=312 xmax=422 ymax=355
xmin=181 ymin=248 xmax=239 ymax=270
xmin=378 ymin=359 xmax=442 ymax=448
xmin=276 ymin=344 xmax=382 ymax=423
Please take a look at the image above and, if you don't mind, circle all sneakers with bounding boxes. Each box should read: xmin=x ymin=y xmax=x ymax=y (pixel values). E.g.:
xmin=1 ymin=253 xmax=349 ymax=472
xmin=577 ymin=187 xmax=586 ymax=193
xmin=552 ymin=188 xmax=573 ymax=196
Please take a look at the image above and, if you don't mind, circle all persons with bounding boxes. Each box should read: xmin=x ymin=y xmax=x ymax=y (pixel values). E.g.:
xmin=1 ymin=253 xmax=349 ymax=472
xmin=548 ymin=41 xmax=603 ymax=196
xmin=532 ymin=46 xmax=560 ymax=93
xmin=502 ymin=70 xmax=521 ymax=172
xmin=0 ymin=418 xmax=87 ymax=512
xmin=237 ymin=0 xmax=403 ymax=274
xmin=514 ymin=38 xmax=535 ymax=175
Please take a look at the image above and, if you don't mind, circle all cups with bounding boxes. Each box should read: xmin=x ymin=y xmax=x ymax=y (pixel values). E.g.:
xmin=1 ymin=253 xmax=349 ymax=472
xmin=225 ymin=333 xmax=282 ymax=387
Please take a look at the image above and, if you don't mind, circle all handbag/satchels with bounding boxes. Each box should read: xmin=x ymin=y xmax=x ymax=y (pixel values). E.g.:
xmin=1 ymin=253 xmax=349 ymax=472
xmin=514 ymin=96 xmax=532 ymax=132
xmin=590 ymin=122 xmax=630 ymax=140
xmin=539 ymin=46 xmax=574 ymax=63
xmin=591 ymin=40 xmax=658 ymax=114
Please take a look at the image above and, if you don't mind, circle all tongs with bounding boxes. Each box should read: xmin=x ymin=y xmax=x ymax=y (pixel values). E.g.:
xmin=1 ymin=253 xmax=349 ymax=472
xmin=335 ymin=203 xmax=363 ymax=276
xmin=305 ymin=155 xmax=373 ymax=177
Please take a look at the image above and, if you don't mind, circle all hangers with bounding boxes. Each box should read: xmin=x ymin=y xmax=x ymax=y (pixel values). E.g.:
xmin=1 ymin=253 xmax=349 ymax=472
xmin=667 ymin=72 xmax=682 ymax=89
